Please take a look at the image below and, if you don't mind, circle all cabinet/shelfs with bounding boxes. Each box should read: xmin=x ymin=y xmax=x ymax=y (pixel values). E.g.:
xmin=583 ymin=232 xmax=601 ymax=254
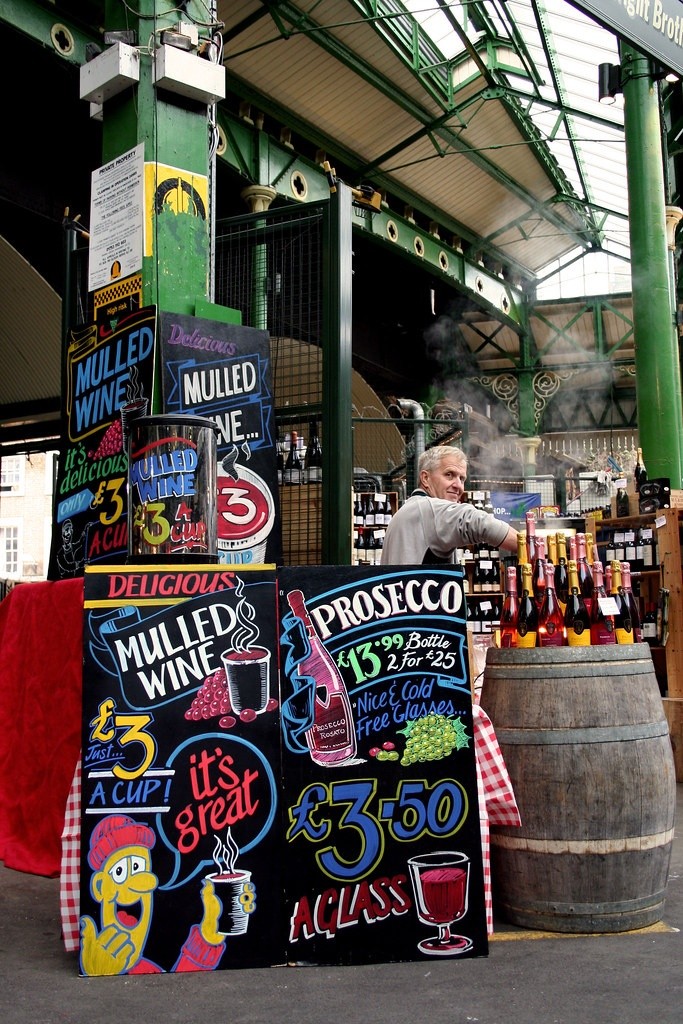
xmin=451 ymin=538 xmax=506 ymax=635
xmin=351 ymin=491 xmax=397 ymax=566
xmin=595 ymin=510 xmax=682 ymax=699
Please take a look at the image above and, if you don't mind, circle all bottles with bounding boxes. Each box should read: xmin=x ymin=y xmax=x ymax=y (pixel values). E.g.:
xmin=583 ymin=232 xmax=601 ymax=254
xmin=274 ymin=426 xmax=284 ymax=486
xmin=284 ymin=431 xmax=302 ymax=487
xmin=633 ymin=447 xmax=648 ymax=493
xmin=616 ymin=472 xmax=630 ymax=518
xmin=304 ymin=421 xmax=323 ymax=483
xmin=354 ymin=490 xmax=663 ymax=648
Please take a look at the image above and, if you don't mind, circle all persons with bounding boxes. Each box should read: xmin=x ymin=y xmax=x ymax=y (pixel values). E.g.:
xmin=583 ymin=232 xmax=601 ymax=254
xmin=381 ymin=445 xmax=523 ymax=571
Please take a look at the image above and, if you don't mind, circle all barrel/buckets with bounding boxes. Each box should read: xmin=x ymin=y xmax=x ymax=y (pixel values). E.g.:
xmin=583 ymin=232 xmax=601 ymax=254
xmin=478 ymin=642 xmax=679 ymax=934
